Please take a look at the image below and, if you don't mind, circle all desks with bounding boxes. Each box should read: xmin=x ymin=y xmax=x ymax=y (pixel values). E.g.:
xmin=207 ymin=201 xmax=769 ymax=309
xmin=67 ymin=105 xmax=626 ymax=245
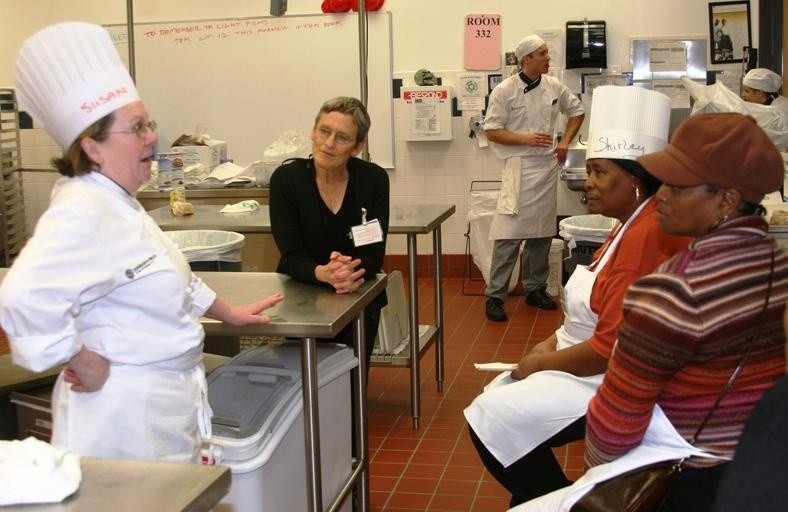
xmin=144 ymin=201 xmax=457 ymax=432
xmin=0 ymin=265 xmax=388 ymax=512
xmin=2 ymin=441 xmax=233 ymax=511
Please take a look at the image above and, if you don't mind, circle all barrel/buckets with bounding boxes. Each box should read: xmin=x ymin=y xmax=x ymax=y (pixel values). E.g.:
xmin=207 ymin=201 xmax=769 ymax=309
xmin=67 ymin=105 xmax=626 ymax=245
xmin=545 ymin=239 xmax=565 ymax=296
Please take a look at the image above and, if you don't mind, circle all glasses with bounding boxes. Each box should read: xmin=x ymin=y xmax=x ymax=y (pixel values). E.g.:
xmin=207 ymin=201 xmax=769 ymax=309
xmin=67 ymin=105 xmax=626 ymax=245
xmin=86 ymin=118 xmax=158 ymax=138
xmin=312 ymin=124 xmax=361 ymax=147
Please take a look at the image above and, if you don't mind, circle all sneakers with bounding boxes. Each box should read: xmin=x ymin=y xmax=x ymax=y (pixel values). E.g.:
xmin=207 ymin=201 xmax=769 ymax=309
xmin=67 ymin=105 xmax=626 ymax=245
xmin=523 ymin=290 xmax=559 ymax=309
xmin=484 ymin=294 xmax=509 ymax=324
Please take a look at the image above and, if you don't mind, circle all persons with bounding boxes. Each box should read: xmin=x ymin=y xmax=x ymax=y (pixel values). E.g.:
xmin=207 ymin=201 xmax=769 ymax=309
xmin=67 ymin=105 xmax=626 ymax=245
xmin=462 ymin=85 xmax=697 ymax=508
xmin=269 ymin=96 xmax=389 ymax=457
xmin=0 ymin=21 xmax=284 ymax=464
xmin=483 ymin=35 xmax=586 ymax=322
xmin=713 ymin=18 xmax=733 ymax=60
xmin=741 ymin=68 xmax=788 ymax=107
xmin=505 ymin=113 xmax=788 ymax=512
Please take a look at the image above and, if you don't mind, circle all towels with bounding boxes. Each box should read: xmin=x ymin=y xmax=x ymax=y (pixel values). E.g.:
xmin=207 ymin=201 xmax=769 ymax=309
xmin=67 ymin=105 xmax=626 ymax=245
xmin=1 ymin=429 xmax=84 ymax=501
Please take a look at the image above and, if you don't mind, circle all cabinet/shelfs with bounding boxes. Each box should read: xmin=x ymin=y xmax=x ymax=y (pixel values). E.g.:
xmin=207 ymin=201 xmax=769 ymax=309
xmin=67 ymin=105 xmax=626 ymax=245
xmin=135 ymin=182 xmax=285 ymax=274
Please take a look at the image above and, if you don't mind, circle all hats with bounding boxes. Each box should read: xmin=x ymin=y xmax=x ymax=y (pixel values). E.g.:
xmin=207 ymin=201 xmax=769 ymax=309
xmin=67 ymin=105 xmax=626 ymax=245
xmin=634 ymin=108 xmax=788 ymax=204
xmin=12 ymin=19 xmax=141 ymax=155
xmin=741 ymin=67 xmax=784 ymax=94
xmin=514 ymin=34 xmax=546 ymax=61
xmin=583 ymin=80 xmax=675 ymax=167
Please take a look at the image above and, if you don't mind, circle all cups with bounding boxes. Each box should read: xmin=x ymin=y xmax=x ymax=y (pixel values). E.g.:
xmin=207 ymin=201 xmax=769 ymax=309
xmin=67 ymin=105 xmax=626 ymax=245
xmin=170 ymin=187 xmax=185 ymax=210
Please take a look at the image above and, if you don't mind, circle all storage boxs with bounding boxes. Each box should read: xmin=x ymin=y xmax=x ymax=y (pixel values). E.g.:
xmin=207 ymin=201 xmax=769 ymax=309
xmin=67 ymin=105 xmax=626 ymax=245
xmin=156 ymin=139 xmax=228 ymax=188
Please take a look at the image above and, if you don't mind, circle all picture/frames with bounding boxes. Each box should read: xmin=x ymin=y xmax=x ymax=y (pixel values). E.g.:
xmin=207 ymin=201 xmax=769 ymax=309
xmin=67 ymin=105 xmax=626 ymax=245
xmin=706 ymin=2 xmax=752 ymax=65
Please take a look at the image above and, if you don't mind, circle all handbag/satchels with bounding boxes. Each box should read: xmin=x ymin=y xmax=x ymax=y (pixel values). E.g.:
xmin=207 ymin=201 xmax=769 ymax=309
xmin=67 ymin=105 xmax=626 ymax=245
xmin=563 ymin=442 xmax=699 ymax=512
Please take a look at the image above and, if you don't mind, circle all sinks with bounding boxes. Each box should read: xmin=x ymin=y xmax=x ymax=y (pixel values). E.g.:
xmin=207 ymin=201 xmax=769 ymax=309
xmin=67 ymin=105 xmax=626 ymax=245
xmin=562 ymin=167 xmax=588 ymax=174
xmin=560 ymin=174 xmax=589 ymax=191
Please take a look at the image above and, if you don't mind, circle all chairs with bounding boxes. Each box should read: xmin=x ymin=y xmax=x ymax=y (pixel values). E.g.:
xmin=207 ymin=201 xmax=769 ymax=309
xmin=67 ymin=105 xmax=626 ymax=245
xmin=708 ymin=374 xmax=788 ymax=511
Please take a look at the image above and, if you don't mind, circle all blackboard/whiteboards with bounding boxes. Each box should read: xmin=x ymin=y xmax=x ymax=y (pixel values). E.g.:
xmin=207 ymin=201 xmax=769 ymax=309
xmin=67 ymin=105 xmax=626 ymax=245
xmin=101 ymin=11 xmax=397 ymax=170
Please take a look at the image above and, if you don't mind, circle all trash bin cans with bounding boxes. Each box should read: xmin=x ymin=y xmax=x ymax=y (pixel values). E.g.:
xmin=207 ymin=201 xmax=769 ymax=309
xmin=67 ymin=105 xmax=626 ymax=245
xmin=559 ymin=214 xmax=621 ymax=287
xmin=161 ymin=229 xmax=245 ymax=272
